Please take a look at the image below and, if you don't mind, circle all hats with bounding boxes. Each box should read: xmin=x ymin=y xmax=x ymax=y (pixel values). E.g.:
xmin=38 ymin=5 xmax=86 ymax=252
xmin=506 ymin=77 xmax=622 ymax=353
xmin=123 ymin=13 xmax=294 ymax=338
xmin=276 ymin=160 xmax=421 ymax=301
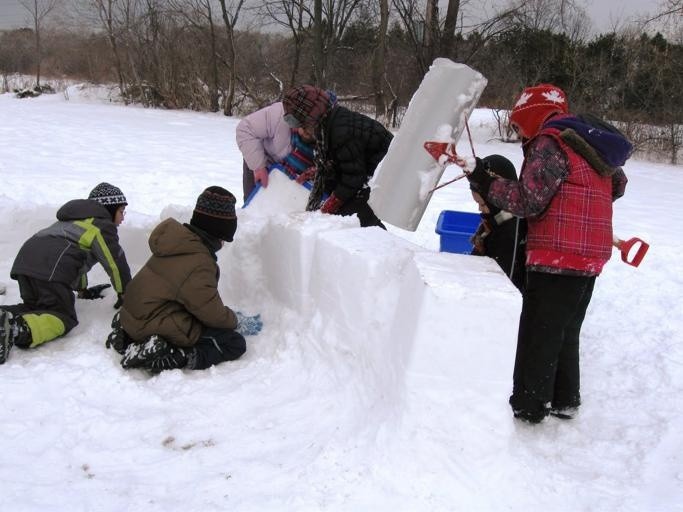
xmin=90 ymin=182 xmax=128 ymax=205
xmin=190 ymin=185 xmax=237 ymax=243
xmin=283 ymin=84 xmax=337 ymax=133
xmin=510 ymin=82 xmax=569 ymax=139
xmin=469 ymin=154 xmax=517 ymax=217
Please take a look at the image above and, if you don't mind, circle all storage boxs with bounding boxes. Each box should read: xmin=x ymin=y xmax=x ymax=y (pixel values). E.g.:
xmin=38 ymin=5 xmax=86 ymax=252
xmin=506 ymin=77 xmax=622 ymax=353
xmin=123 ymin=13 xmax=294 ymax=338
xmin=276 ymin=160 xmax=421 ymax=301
xmin=434 ymin=210 xmax=480 ymax=255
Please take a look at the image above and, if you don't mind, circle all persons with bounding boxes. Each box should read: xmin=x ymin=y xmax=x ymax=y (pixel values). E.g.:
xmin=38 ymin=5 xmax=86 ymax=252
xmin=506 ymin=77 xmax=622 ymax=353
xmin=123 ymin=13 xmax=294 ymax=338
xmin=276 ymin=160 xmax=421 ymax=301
xmin=0 ymin=182 xmax=130 ymax=367
xmin=103 ymin=185 xmax=265 ymax=374
xmin=235 ymin=87 xmax=337 ymax=206
xmin=282 ymin=83 xmax=394 ymax=217
xmin=453 ymin=82 xmax=635 ymax=425
xmin=467 ymin=153 xmax=529 ymax=292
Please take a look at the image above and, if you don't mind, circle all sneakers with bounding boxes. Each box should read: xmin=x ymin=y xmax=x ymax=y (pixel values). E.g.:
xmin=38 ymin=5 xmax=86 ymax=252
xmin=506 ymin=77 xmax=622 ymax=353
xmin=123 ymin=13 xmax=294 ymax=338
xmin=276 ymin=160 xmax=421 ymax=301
xmin=551 ymin=405 xmax=578 ymax=419
xmin=111 ymin=312 xmax=130 ymax=354
xmin=120 ymin=335 xmax=172 ymax=367
xmin=1 ymin=309 xmax=20 ymax=363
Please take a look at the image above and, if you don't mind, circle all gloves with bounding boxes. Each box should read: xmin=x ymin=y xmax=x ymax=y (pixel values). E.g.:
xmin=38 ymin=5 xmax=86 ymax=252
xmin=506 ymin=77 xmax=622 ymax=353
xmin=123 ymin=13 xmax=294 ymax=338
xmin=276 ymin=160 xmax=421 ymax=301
xmin=115 ymin=293 xmax=129 ymax=308
xmin=80 ymin=285 xmax=112 ymax=301
xmin=296 ymin=168 xmax=317 ymax=184
xmin=254 ymin=168 xmax=269 ymax=187
xmin=235 ymin=311 xmax=263 ymax=336
xmin=320 ymin=192 xmax=342 ymax=212
xmin=464 ymin=167 xmax=498 ymax=197
